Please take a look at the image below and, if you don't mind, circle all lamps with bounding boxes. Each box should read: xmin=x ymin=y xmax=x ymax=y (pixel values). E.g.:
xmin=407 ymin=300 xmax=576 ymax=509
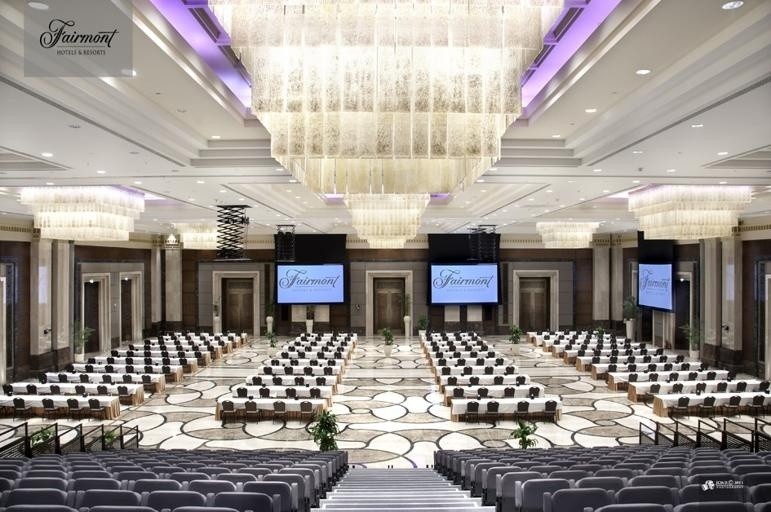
xmin=628 ymin=184 xmax=750 ymax=240
xmin=172 ymin=221 xmax=223 ymax=251
xmin=206 ymin=0 xmax=568 ymax=193
xmin=20 ymin=186 xmax=145 ymax=242
xmin=535 ymin=219 xmax=598 ymax=249
xmin=322 ymin=184 xmax=449 ymax=249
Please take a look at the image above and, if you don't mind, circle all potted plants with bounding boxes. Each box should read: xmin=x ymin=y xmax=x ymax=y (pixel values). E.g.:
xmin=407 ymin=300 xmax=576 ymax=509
xmin=623 ymin=296 xmax=635 ymax=339
xmin=403 ymin=294 xmax=410 ymax=336
xmin=266 ymin=299 xmax=275 ymax=333
xmin=306 ymin=304 xmax=315 ymax=333
xmin=382 ymin=327 xmax=393 ymax=345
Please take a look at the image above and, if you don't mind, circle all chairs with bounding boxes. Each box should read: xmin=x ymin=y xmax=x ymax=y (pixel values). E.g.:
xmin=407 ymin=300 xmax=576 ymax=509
xmin=0 ymin=329 xmax=247 ymax=423
xmin=526 ymin=328 xmax=771 ymax=421
xmin=426 ymin=330 xmax=557 ymax=425
xmin=219 ymin=332 xmax=355 ymax=427
